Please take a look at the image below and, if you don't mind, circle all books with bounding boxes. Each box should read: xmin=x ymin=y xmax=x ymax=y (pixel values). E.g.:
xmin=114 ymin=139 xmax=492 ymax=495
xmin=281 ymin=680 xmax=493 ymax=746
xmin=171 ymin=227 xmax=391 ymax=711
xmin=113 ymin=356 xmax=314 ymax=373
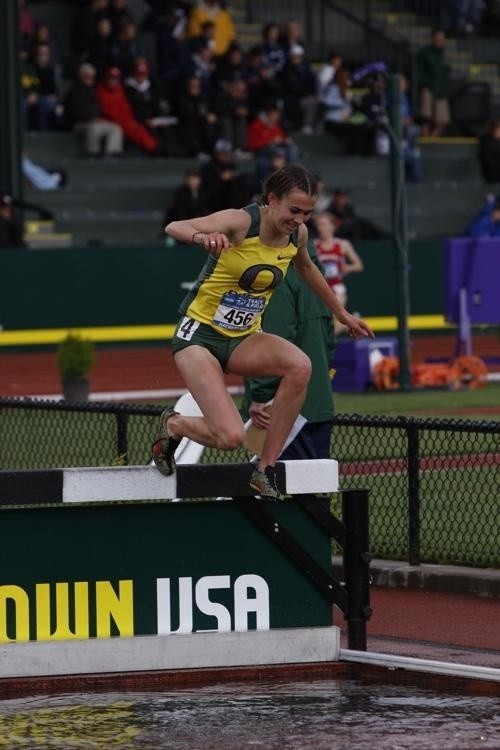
xmin=244 ymin=396 xmax=307 ymax=458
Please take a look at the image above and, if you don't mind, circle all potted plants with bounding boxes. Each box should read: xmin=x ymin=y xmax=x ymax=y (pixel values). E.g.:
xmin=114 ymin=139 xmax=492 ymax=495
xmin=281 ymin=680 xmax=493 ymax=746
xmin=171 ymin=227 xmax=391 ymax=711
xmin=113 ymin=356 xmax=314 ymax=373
xmin=57 ymin=334 xmax=96 ymax=401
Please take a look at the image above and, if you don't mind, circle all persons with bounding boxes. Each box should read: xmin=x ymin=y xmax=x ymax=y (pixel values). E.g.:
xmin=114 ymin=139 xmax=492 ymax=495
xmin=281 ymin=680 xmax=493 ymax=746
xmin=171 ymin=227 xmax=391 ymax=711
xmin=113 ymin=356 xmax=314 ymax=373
xmin=307 ymin=211 xmax=365 ymax=319
xmin=150 ymin=165 xmax=379 ymax=504
xmin=238 ymin=239 xmax=338 ymax=459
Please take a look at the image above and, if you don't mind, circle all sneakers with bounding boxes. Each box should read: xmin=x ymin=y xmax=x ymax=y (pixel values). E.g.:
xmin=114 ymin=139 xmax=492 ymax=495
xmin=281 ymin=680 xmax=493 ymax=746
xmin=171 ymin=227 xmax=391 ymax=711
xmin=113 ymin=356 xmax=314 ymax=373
xmin=152 ymin=409 xmax=182 ymax=476
xmin=249 ymin=465 xmax=287 ymax=502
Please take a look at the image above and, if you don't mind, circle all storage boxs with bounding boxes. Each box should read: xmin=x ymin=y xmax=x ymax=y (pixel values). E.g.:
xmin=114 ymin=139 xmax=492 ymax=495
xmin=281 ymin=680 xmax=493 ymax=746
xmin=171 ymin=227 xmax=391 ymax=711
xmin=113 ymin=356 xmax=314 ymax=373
xmin=331 ymin=337 xmax=400 ymax=393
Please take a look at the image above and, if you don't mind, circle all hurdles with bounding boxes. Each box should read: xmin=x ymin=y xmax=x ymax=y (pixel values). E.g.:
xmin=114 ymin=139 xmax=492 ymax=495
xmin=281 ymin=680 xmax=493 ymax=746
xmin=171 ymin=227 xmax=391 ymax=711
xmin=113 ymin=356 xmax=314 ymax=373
xmin=0 ymin=458 xmax=372 ymax=650
